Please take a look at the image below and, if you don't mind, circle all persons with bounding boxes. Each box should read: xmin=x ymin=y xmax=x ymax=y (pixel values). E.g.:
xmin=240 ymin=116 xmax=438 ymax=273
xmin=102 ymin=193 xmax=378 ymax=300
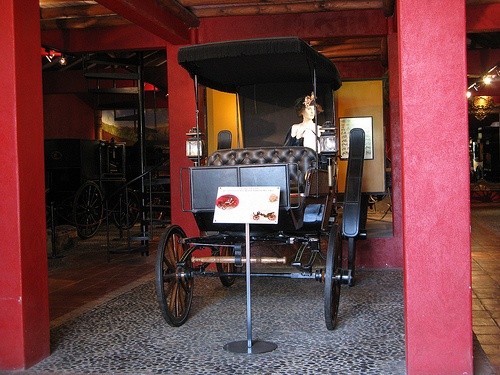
xmin=284 ymin=97 xmax=326 ymax=155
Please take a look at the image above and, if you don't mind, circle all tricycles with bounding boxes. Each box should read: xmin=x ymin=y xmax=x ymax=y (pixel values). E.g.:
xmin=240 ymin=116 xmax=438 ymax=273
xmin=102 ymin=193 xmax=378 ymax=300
xmin=155 ymin=36 xmax=368 ymax=332
xmin=43 ymin=138 xmax=141 ymax=239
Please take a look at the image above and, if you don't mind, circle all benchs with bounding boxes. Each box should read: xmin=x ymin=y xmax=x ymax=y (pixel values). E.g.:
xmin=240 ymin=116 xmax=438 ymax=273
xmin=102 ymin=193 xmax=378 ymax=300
xmin=207 ymin=146 xmax=317 ymax=205
